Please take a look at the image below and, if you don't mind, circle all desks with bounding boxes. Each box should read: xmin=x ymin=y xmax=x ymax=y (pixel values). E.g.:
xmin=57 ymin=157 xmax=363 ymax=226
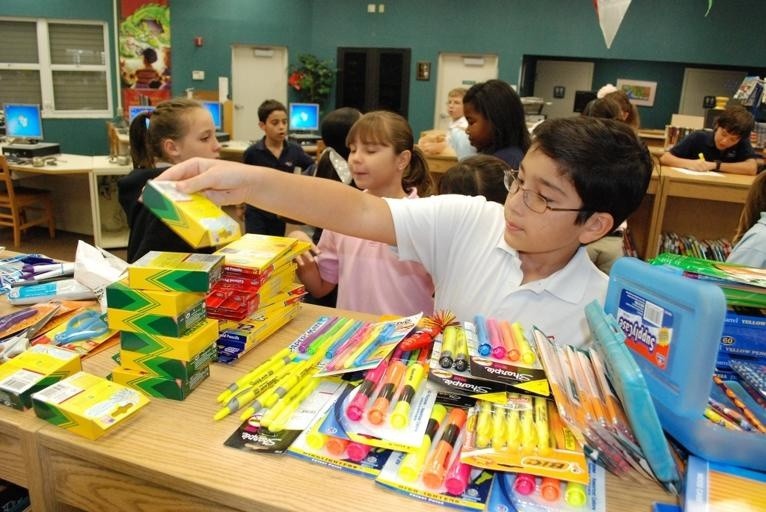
xmin=36 ymin=300 xmax=766 ymax=512
xmin=218 ymin=141 xmax=326 ymax=165
xmin=625 ymin=129 xmax=757 ymax=261
xmin=0 ymin=249 xmax=122 ymax=512
xmin=0 ymin=152 xmax=98 ymax=250
xmin=414 ymin=128 xmax=459 ymax=174
xmin=92 ymin=156 xmax=135 ymax=250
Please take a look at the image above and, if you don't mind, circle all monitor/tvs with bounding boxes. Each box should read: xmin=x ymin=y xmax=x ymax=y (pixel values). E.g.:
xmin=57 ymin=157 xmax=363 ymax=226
xmin=288 ymin=102 xmax=320 ymax=135
xmin=129 ymin=106 xmax=156 ymax=129
xmin=204 ymin=102 xmax=222 ymax=131
xmin=574 ymin=91 xmax=597 ymax=112
xmin=3 ymin=103 xmax=44 ymax=144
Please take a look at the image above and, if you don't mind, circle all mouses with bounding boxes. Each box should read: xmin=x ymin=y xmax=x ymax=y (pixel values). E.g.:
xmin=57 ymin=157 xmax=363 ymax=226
xmin=17 ymin=160 xmax=26 ymax=164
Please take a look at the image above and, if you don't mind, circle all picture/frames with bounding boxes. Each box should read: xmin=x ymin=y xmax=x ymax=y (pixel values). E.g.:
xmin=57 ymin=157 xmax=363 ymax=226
xmin=616 ymin=78 xmax=659 ymax=108
xmin=414 ymin=60 xmax=431 ymax=81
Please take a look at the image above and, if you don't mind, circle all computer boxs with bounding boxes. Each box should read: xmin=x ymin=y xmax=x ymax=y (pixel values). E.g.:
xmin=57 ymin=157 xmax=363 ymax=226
xmin=3 ymin=142 xmax=59 ymax=158
xmin=215 ymin=132 xmax=229 ymax=141
xmin=288 ymin=135 xmax=323 ymax=146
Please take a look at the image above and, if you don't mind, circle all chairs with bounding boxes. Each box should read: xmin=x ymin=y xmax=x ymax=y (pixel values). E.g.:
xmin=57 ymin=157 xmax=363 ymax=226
xmin=0 ymin=155 xmax=56 ymax=249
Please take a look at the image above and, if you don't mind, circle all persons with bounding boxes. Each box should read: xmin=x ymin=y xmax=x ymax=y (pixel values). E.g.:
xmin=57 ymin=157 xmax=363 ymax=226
xmin=423 ymin=86 xmax=478 ymax=164
xmin=604 ymin=89 xmax=641 ymax=130
xmin=658 ymin=104 xmax=759 ymax=175
xmin=116 ymin=97 xmax=222 ymax=268
xmin=230 ymin=99 xmax=316 ymax=240
xmin=436 ymin=152 xmax=515 ymax=206
xmin=723 ymin=169 xmax=766 ymax=270
xmin=120 ymin=48 xmax=171 ymax=89
xmin=299 ymin=107 xmax=364 ymax=310
xmin=286 ymin=110 xmax=441 ymax=318
xmin=135 ymin=113 xmax=657 ymax=357
xmin=462 ymin=79 xmax=531 ymax=175
xmin=582 ymin=97 xmax=627 ymax=274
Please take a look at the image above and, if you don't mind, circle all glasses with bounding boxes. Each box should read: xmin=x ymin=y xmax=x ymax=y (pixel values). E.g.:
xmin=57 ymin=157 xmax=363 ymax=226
xmin=501 ymin=167 xmax=597 ymax=215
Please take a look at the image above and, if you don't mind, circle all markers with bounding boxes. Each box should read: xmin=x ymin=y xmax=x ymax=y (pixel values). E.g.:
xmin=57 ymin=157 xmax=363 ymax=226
xmin=212 ymin=316 xmax=689 ymax=508
xmin=10 ymin=256 xmax=75 ymax=288
xmin=698 ymin=152 xmax=709 ymax=173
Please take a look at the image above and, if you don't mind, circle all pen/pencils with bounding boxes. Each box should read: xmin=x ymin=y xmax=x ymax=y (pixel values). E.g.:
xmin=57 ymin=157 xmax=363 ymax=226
xmin=704 ymin=372 xmax=766 ymax=435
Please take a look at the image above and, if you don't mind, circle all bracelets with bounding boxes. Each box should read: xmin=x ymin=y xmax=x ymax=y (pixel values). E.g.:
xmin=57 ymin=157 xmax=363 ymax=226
xmin=235 ymin=205 xmax=244 ymax=209
xmin=713 ymin=158 xmax=721 ymax=171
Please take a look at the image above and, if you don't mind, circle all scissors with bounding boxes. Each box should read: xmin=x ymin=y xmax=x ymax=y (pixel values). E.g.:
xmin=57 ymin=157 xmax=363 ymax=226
xmin=0 ymin=305 xmax=61 ymax=362
xmin=47 ymin=311 xmax=108 ymax=347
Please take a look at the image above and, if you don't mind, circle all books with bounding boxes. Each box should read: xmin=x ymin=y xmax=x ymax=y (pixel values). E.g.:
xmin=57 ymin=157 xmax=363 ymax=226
xmin=664 ymin=68 xmax=766 ymax=153
xmin=625 ymin=228 xmax=731 ymax=264
